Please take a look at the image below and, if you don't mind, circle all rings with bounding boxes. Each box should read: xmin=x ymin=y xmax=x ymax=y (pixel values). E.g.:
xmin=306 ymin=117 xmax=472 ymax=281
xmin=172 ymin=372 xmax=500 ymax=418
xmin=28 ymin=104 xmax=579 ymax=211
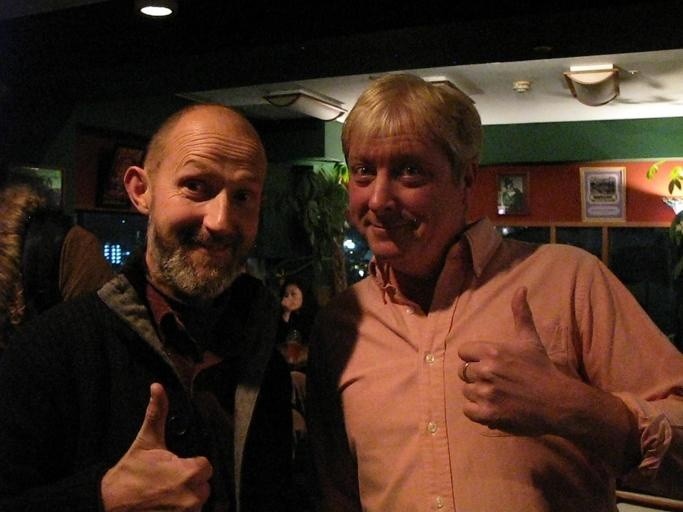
xmin=462 ymin=360 xmax=476 ymax=383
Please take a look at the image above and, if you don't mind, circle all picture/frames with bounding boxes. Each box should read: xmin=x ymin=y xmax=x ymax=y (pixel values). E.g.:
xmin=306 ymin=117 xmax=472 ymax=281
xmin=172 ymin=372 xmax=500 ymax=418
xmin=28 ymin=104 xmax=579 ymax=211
xmin=495 ymin=171 xmax=530 ymax=216
xmin=578 ymin=166 xmax=627 ymax=224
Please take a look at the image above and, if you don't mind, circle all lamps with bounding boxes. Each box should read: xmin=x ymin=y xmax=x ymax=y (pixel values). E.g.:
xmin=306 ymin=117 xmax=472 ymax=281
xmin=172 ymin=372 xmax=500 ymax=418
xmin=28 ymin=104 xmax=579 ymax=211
xmin=562 ymin=62 xmax=620 ymax=108
xmin=262 ymin=90 xmax=348 ymax=122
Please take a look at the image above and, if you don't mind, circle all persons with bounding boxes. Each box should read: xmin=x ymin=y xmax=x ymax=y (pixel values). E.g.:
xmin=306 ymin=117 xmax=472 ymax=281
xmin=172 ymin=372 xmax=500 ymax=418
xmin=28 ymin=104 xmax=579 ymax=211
xmin=503 ymin=176 xmax=523 ymax=215
xmin=0 ymin=105 xmax=294 ymax=510
xmin=0 ymin=165 xmax=114 ymax=332
xmin=280 ymin=277 xmax=319 ymax=346
xmin=306 ymin=74 xmax=683 ymax=511
